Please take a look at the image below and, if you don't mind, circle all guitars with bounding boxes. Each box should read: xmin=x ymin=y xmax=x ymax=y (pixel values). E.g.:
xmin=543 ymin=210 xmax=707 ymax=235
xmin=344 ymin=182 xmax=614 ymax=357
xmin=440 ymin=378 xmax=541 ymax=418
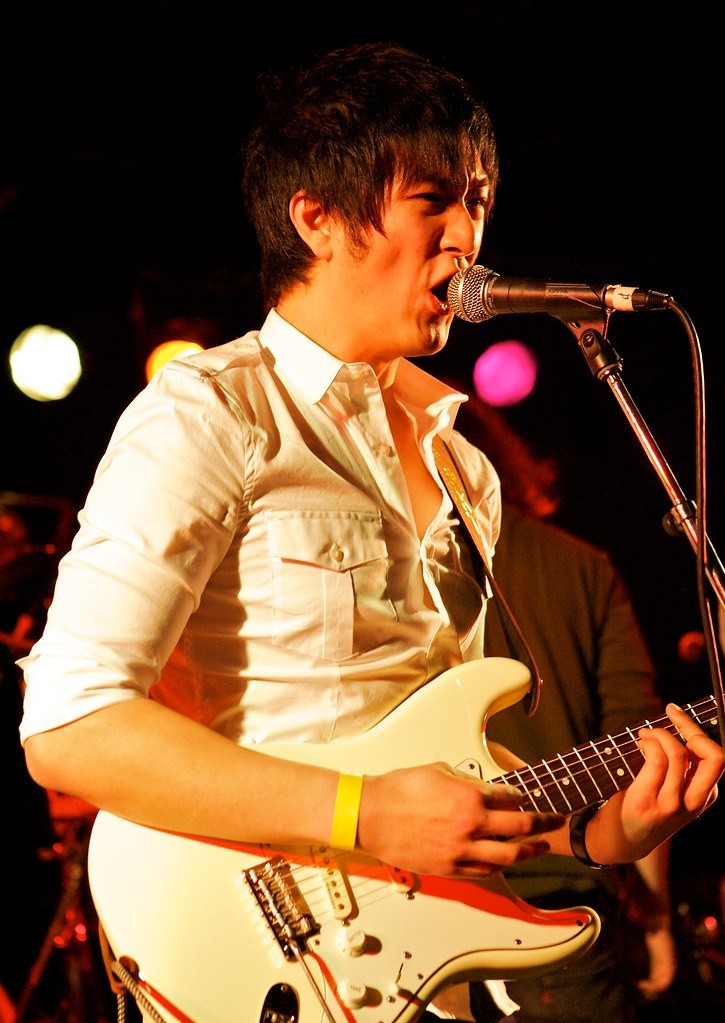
xmin=84 ymin=655 xmax=723 ymax=1023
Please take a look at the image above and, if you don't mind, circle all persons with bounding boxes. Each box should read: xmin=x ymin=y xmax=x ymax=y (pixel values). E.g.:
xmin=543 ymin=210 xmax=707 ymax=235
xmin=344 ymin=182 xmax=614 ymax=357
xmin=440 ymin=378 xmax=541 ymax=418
xmin=433 ymin=369 xmax=679 ymax=1021
xmin=15 ymin=76 xmax=725 ymax=1022
xmin=0 ymin=512 xmax=69 ymax=1023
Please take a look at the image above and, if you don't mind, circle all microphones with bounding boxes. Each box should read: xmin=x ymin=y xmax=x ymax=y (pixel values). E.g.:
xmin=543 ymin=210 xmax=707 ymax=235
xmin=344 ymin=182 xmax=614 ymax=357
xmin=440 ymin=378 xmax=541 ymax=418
xmin=447 ymin=264 xmax=674 ymax=323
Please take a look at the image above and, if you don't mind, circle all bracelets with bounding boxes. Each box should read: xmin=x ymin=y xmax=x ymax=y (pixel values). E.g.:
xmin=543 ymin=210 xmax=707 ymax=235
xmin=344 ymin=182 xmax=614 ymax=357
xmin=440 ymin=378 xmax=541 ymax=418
xmin=325 ymin=766 xmax=368 ymax=853
xmin=570 ymin=793 xmax=611 ymax=873
